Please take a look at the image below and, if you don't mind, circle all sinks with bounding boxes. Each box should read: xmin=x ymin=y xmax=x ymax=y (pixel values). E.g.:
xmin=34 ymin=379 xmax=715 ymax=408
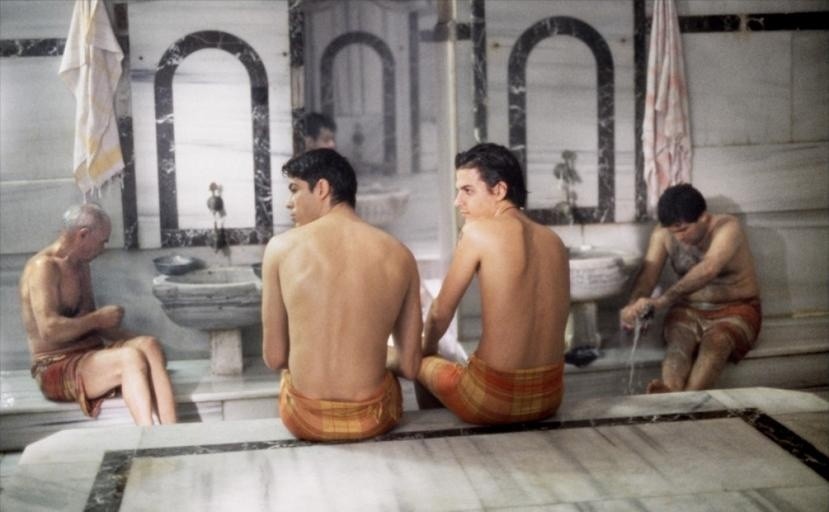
xmin=148 ymin=265 xmax=267 ymax=333
xmin=566 ymin=245 xmax=629 ymax=304
xmin=355 ymin=185 xmax=407 ymax=229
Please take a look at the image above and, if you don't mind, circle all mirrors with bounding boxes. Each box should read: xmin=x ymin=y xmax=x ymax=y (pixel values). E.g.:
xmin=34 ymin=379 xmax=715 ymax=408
xmin=288 ymin=0 xmax=487 ymax=272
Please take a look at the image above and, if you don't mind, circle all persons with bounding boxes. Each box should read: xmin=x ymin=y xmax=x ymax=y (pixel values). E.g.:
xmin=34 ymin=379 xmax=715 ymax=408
xmin=18 ymin=202 xmax=178 ymax=425
xmin=262 ymin=146 xmax=422 ymax=444
xmin=619 ymin=179 xmax=763 ymax=397
xmin=417 ymin=142 xmax=571 ymax=422
xmin=295 ymin=113 xmax=339 ymax=152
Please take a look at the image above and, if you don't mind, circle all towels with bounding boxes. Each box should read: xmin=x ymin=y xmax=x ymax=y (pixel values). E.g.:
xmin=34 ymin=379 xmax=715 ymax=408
xmin=640 ymin=0 xmax=695 ymax=220
xmin=57 ymin=0 xmax=125 ymax=205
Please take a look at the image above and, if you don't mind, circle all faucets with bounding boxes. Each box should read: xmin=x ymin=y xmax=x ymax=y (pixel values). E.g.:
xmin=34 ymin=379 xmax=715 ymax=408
xmin=209 ymin=221 xmax=224 ymax=251
xmin=556 ymin=202 xmax=576 ymax=230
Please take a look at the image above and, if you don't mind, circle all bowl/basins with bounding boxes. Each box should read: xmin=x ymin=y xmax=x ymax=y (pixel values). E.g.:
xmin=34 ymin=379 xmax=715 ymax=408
xmin=153 ymin=254 xmax=194 ymax=275
